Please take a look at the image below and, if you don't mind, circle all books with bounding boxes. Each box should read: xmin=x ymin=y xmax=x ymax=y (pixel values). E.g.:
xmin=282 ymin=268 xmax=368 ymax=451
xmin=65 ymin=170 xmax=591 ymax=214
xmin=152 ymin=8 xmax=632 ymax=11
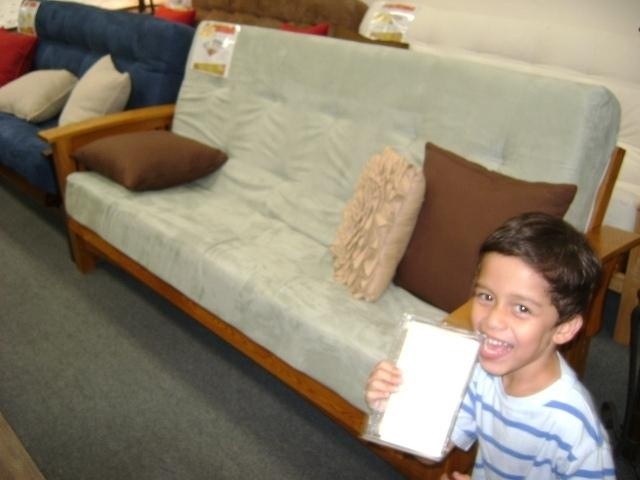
xmin=376 ymin=320 xmax=481 ymax=461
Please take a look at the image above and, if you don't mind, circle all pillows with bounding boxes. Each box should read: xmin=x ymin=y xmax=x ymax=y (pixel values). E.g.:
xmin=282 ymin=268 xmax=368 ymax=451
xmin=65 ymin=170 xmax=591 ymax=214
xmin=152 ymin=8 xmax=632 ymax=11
xmin=57 ymin=53 xmax=132 ymax=128
xmin=330 ymin=145 xmax=427 ymax=304
xmin=0 ymin=25 xmax=39 ymax=88
xmin=0 ymin=68 xmax=78 ymax=124
xmin=392 ymin=141 xmax=579 ymax=315
xmin=67 ymin=127 xmax=229 ymax=193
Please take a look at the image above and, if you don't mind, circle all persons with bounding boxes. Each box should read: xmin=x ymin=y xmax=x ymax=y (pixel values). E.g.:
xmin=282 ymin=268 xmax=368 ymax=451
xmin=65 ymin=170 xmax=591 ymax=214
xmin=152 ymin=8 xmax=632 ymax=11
xmin=364 ymin=212 xmax=616 ymax=480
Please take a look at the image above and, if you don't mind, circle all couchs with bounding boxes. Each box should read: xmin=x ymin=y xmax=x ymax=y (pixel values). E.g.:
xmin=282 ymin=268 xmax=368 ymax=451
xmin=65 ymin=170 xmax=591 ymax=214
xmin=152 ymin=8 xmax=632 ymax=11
xmin=36 ymin=20 xmax=640 ymax=479
xmin=0 ymin=0 xmax=194 ymax=213
